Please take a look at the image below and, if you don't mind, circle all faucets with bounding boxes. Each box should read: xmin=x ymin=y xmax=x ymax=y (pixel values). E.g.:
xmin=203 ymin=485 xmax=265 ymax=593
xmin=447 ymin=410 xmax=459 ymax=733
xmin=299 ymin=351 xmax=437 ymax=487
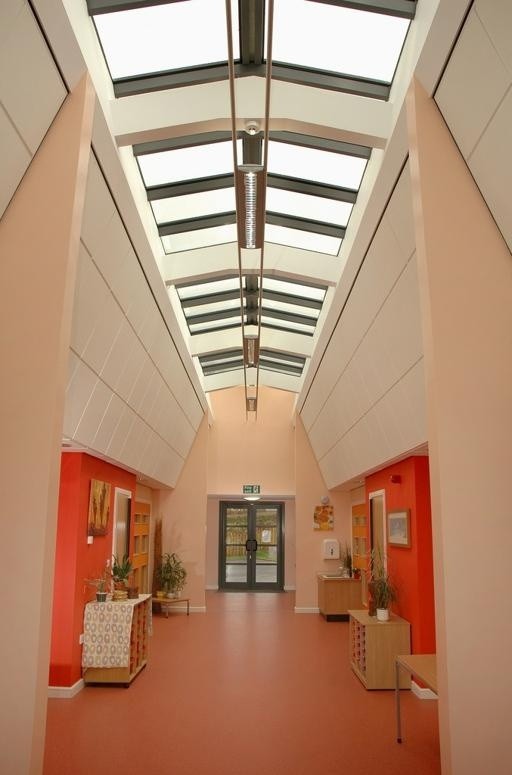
xmin=339 ymin=566 xmax=344 ymax=576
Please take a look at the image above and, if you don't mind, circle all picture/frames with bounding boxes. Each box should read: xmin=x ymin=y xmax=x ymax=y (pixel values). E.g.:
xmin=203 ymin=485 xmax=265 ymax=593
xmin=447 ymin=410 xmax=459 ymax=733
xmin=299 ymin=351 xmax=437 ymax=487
xmin=386 ymin=510 xmax=410 ymax=549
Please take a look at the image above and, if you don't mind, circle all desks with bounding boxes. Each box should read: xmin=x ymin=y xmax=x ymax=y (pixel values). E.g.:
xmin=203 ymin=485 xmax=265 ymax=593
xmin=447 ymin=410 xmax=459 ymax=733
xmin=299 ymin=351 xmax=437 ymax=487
xmin=152 ymin=597 xmax=190 ymax=618
xmin=83 ymin=594 xmax=152 ymax=686
xmin=347 ymin=609 xmax=412 ymax=690
xmin=395 ymin=654 xmax=438 ymax=744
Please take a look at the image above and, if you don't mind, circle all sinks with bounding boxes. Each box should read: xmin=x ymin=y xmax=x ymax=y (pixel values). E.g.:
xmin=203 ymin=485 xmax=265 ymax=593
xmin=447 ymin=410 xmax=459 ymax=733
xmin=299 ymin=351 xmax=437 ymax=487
xmin=321 ymin=573 xmax=349 ymax=578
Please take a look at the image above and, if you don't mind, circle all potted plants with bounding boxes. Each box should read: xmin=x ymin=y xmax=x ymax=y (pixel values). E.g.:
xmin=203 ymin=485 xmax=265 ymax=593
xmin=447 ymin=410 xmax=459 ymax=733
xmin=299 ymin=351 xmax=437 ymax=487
xmin=154 ymin=552 xmax=187 ymax=599
xmin=83 ymin=552 xmax=134 ymax=602
xmin=358 ymin=531 xmax=399 ymax=621
